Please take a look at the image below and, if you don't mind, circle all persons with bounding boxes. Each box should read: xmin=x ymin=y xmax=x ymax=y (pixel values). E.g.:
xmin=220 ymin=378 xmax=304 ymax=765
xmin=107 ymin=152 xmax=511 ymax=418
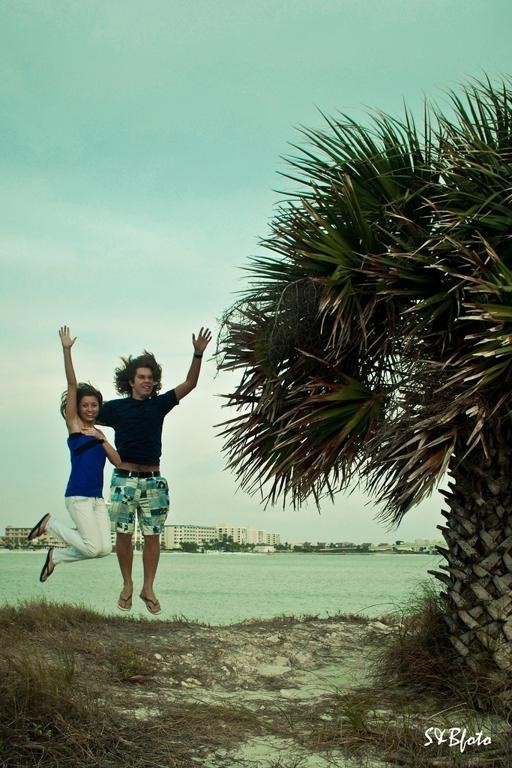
xmin=96 ymin=326 xmax=212 ymax=614
xmin=28 ymin=324 xmax=121 ymax=582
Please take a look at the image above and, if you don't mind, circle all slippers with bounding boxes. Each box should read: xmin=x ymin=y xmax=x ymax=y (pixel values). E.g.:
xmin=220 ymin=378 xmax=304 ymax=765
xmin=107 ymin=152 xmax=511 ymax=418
xmin=118 ymin=594 xmax=133 ymax=610
xmin=140 ymin=594 xmax=161 ymax=614
xmin=39 ymin=548 xmax=55 ymax=582
xmin=28 ymin=513 xmax=51 ymax=540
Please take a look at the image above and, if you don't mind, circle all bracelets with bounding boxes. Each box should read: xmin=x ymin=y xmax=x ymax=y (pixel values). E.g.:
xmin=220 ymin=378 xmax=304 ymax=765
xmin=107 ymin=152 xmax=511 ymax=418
xmin=193 ymin=353 xmax=203 ymax=358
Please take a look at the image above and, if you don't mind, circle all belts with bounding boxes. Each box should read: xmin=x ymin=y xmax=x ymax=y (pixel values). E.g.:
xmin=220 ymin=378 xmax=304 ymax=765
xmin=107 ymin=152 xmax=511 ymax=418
xmin=114 ymin=466 xmax=161 ymax=479
